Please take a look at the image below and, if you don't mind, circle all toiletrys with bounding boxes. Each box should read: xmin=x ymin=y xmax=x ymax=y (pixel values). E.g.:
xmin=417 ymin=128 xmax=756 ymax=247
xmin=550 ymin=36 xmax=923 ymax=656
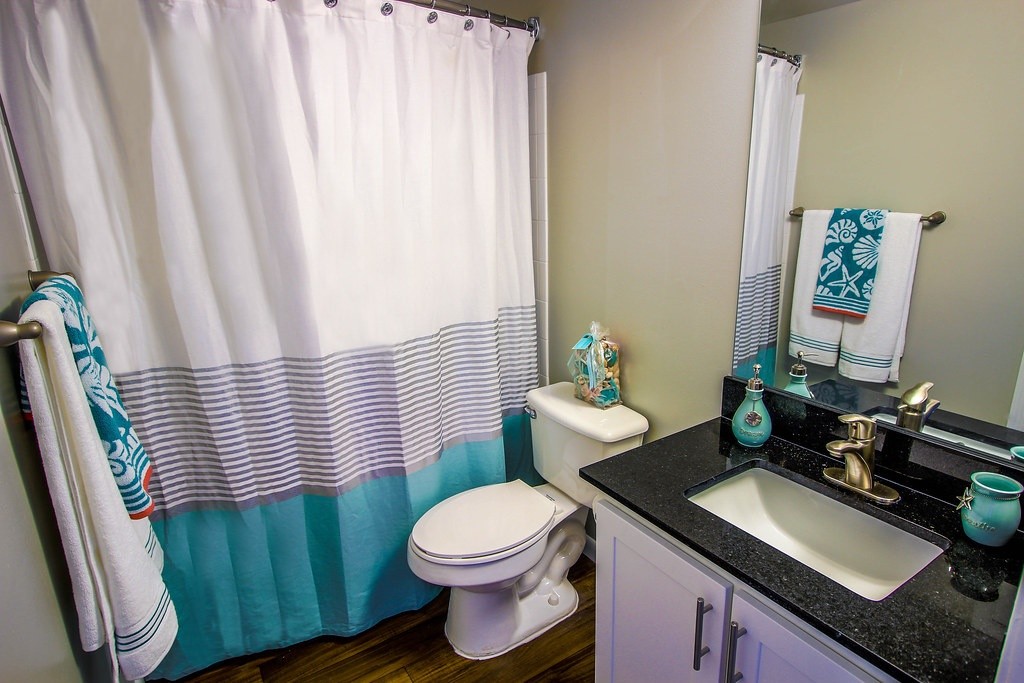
xmin=783 ymin=350 xmax=820 ymax=400
xmin=732 ymin=364 xmax=772 ymax=447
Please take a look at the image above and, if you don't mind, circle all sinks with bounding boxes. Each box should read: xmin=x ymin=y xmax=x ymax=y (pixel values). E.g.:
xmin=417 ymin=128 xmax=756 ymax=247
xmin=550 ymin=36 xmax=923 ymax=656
xmin=683 ymin=459 xmax=952 ymax=602
xmin=859 ymin=403 xmax=1019 ymax=463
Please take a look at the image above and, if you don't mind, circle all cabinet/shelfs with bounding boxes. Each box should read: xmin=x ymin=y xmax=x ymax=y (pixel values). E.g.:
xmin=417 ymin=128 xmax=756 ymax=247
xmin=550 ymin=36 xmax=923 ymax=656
xmin=592 ymin=491 xmax=901 ymax=683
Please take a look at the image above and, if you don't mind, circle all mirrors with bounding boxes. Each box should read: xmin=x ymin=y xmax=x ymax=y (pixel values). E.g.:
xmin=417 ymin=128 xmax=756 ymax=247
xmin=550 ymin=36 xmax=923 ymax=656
xmin=730 ymin=0 xmax=1024 ymax=450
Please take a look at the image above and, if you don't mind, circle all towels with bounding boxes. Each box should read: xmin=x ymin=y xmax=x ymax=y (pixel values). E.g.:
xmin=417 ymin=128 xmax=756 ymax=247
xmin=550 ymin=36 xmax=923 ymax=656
xmin=789 ymin=207 xmax=923 ymax=384
xmin=17 ymin=274 xmax=179 ymax=681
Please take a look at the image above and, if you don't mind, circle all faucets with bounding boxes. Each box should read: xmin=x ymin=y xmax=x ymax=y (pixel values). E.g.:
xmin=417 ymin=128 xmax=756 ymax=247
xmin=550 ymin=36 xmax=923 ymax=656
xmin=823 ymin=414 xmax=878 ymax=505
xmin=894 ymin=381 xmax=942 ymax=433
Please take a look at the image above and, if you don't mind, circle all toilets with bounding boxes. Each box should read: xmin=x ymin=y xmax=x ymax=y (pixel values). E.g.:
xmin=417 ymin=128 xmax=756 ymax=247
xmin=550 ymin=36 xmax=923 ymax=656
xmin=408 ymin=381 xmax=649 ymax=660
